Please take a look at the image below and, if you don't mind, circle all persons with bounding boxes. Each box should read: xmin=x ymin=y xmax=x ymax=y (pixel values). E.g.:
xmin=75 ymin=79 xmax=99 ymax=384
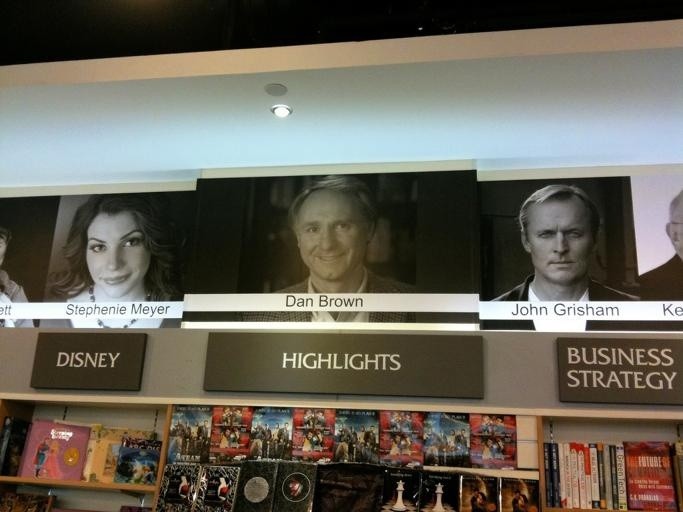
xmin=482 ymin=184 xmax=683 ymax=331
xmin=0 ymin=193 xmax=181 ymax=328
xmin=235 ymin=175 xmax=417 ymax=322
